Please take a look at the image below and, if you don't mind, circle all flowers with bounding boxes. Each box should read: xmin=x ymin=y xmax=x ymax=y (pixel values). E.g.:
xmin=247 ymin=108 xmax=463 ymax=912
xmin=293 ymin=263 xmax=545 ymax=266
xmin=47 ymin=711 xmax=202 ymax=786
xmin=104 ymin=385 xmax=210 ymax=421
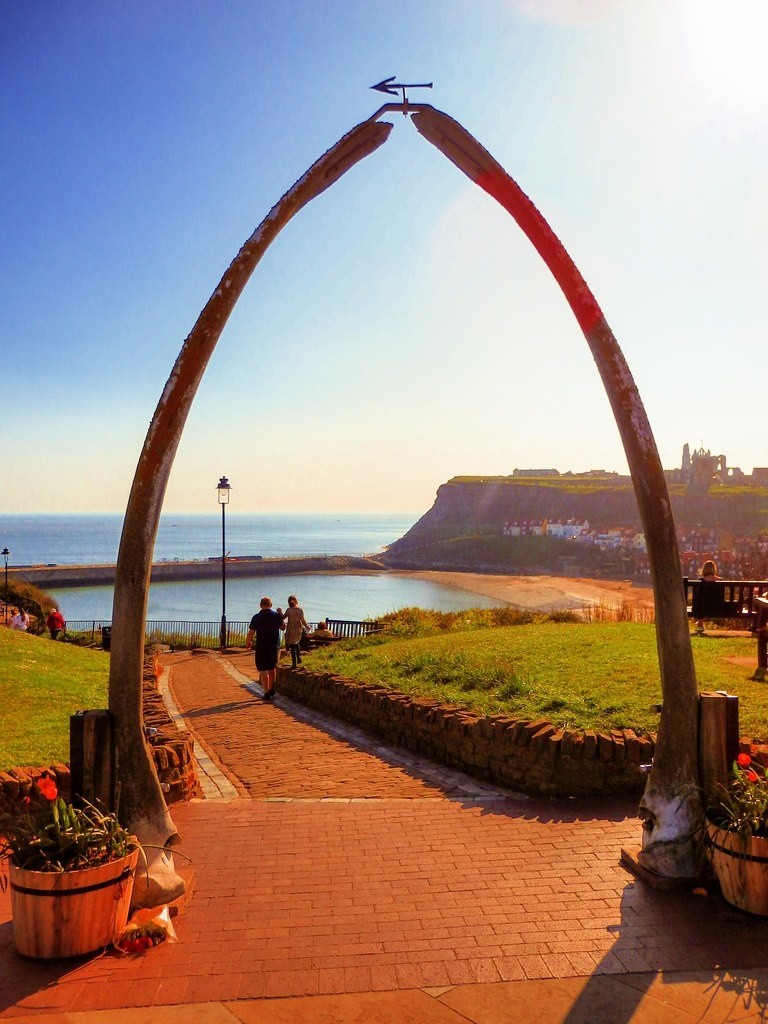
xmin=706 ymin=741 xmax=768 ymax=832
xmin=4 ymin=774 xmax=141 ymax=864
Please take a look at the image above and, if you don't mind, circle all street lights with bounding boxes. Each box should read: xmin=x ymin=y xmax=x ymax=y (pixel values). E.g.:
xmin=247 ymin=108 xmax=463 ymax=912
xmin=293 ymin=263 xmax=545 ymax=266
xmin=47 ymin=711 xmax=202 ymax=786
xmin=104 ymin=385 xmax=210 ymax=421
xmin=1 ymin=546 xmax=11 ymax=626
xmin=214 ymin=475 xmax=234 ymax=651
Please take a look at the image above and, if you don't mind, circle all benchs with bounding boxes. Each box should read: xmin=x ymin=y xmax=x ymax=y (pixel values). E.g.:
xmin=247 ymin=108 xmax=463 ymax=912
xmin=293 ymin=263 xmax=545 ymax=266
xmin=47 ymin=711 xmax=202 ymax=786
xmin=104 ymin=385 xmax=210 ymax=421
xmin=304 ymin=637 xmax=343 ymax=652
xmin=682 ymin=575 xmax=768 ymax=679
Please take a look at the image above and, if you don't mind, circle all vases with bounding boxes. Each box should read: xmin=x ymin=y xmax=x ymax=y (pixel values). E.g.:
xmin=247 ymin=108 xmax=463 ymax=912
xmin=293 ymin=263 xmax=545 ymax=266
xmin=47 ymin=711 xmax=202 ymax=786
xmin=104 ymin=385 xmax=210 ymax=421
xmin=704 ymin=818 xmax=768 ymax=914
xmin=6 ymin=841 xmax=143 ymax=957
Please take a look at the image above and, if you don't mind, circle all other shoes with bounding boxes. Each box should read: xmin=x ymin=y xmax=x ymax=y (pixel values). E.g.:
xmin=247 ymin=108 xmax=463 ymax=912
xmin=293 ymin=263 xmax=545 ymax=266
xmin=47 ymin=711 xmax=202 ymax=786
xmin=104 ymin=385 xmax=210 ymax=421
xmin=297 ymin=658 xmax=301 ymax=664
xmin=694 ymin=621 xmax=704 ymax=631
xmin=261 ymin=688 xmax=275 ymax=700
xmin=289 ymin=664 xmax=296 ymax=669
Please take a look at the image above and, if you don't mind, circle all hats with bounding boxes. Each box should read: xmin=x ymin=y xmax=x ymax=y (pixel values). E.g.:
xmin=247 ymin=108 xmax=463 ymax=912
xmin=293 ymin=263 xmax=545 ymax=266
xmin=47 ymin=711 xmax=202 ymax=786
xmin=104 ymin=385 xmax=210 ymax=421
xmin=50 ymin=608 xmax=57 ymax=613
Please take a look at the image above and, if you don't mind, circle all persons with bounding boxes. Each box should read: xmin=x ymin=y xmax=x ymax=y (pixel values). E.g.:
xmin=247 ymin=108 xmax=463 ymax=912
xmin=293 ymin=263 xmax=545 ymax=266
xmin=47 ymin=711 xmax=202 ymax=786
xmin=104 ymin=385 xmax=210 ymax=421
xmin=277 ymin=596 xmax=310 ymax=669
xmin=308 ymin=620 xmax=334 ymax=647
xmin=7 ymin=609 xmax=17 ymax=629
xmin=13 ymin=607 xmax=29 ymax=632
xmin=246 ymin=597 xmax=286 ymax=700
xmin=47 ymin=608 xmax=63 ymax=641
xmin=692 ymin=561 xmax=724 ymax=634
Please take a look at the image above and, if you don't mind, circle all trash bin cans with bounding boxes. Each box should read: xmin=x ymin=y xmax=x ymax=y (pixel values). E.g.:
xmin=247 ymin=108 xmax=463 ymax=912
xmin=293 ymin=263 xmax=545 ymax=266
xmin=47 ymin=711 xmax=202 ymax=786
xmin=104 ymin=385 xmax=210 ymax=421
xmin=102 ymin=627 xmax=111 ymax=650
